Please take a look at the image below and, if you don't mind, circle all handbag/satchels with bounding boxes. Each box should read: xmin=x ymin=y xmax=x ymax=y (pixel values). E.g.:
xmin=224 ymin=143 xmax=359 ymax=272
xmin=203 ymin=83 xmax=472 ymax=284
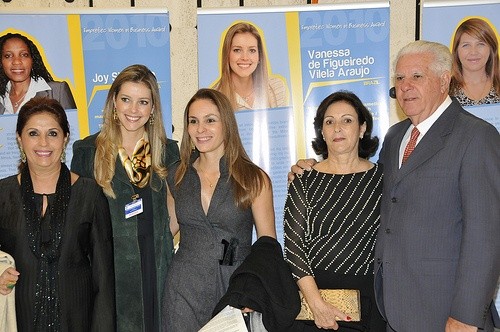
xmin=296 ymin=289 xmax=361 ymax=322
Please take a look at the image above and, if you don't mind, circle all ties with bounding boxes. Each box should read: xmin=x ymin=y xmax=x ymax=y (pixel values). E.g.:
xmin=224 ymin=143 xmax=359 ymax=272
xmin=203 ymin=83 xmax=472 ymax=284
xmin=401 ymin=128 xmax=420 ymax=166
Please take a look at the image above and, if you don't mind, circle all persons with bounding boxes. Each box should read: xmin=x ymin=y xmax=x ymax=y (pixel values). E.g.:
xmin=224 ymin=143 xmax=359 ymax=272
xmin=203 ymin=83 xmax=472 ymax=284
xmin=71 ymin=65 xmax=183 ymax=332
xmin=288 ymin=41 xmax=500 ymax=332
xmin=209 ymin=21 xmax=290 ymax=112
xmin=1 ymin=32 xmax=78 ymax=117
xmin=449 ymin=17 xmax=500 ymax=107
xmin=0 ymin=96 xmax=117 ymax=332
xmin=161 ymin=87 xmax=277 ymax=332
xmin=283 ymin=90 xmax=389 ymax=332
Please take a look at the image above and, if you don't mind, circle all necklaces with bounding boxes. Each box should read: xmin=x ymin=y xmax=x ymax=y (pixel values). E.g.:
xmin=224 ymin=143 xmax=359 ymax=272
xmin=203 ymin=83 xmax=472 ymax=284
xmin=9 ymin=91 xmax=28 ymax=108
xmin=460 ymin=76 xmax=489 ymax=106
xmin=196 ymin=160 xmax=222 ymax=188
xmin=236 ymin=89 xmax=255 ymax=104
